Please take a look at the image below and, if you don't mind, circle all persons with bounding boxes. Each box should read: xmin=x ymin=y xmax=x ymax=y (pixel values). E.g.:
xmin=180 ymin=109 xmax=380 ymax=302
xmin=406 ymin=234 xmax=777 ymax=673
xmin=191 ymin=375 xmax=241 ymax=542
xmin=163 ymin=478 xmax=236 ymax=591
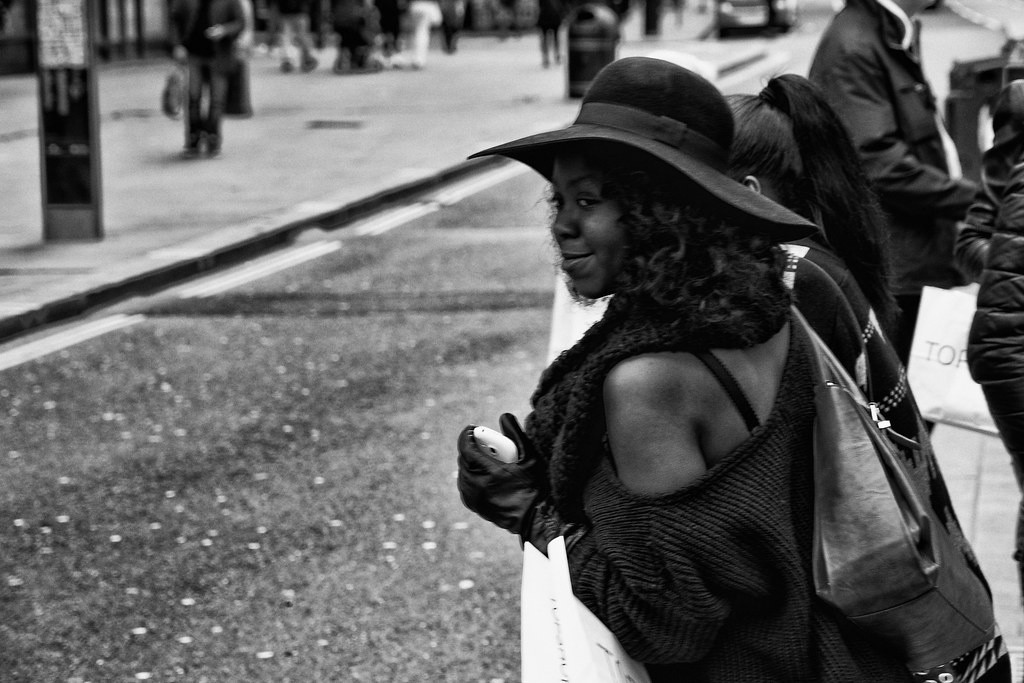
xmin=274 ymin=0 xmax=465 ymax=73
xmin=168 ymin=0 xmax=246 ymax=158
xmin=458 ymin=0 xmax=1024 ymax=683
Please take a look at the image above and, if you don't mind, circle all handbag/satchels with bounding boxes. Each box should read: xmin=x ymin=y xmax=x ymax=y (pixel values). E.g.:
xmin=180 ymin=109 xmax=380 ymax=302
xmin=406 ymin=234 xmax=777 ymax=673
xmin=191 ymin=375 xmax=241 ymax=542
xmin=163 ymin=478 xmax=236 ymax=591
xmin=520 ymin=533 xmax=649 ymax=682
xmin=783 ymin=300 xmax=996 ymax=674
xmin=904 ymin=281 xmax=1005 ymax=439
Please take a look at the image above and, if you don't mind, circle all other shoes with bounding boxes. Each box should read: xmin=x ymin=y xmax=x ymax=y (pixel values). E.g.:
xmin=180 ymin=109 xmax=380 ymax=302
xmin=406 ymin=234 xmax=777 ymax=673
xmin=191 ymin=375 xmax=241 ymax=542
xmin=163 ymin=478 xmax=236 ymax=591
xmin=179 ymin=148 xmax=194 ymax=153
xmin=208 ymin=144 xmax=221 ymax=149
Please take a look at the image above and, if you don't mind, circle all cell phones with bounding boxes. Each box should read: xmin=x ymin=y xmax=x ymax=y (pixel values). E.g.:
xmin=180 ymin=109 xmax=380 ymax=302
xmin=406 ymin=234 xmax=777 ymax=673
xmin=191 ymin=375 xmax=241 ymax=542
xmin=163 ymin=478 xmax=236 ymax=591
xmin=473 ymin=426 xmax=518 ymax=463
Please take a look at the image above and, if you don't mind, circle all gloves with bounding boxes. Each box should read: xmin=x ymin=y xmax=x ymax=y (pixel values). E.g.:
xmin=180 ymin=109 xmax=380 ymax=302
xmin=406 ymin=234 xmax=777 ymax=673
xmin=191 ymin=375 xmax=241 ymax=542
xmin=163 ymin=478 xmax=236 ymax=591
xmin=459 ymin=412 xmax=594 ymax=559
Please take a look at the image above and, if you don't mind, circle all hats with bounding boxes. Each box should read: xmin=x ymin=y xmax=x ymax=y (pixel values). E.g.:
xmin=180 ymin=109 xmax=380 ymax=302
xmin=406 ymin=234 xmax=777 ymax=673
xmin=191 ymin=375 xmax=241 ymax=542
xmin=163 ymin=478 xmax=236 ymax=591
xmin=466 ymin=57 xmax=824 ymax=242
